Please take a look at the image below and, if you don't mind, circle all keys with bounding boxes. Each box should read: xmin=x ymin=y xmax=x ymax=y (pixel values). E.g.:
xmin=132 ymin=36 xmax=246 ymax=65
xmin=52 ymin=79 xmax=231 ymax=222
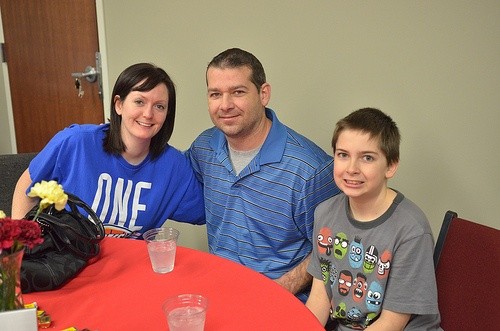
xmin=75 ymin=78 xmax=80 ymax=94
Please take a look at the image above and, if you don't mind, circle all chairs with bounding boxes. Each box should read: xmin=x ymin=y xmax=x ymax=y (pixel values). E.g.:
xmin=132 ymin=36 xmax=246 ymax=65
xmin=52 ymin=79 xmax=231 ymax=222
xmin=433 ymin=210 xmax=500 ymax=331
xmin=0 ymin=152 xmax=39 ymax=217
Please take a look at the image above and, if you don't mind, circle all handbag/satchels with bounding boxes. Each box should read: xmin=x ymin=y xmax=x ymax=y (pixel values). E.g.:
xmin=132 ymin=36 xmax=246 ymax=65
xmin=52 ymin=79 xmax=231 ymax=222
xmin=2 ymin=190 xmax=105 ymax=293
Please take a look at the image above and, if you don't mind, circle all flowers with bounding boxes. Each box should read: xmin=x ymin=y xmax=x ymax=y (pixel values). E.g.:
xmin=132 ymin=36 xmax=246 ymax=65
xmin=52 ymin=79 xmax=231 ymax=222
xmin=0 ymin=180 xmax=68 ymax=312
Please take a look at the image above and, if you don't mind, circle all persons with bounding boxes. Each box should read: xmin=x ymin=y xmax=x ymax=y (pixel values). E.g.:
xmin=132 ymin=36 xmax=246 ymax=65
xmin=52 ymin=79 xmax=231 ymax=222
xmin=184 ymin=47 xmax=343 ymax=304
xmin=304 ymin=107 xmax=443 ymax=331
xmin=11 ymin=62 xmax=205 ymax=240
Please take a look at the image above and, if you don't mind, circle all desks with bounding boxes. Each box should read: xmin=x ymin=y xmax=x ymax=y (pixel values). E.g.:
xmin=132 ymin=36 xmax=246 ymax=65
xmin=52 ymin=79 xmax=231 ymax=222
xmin=23 ymin=236 xmax=327 ymax=331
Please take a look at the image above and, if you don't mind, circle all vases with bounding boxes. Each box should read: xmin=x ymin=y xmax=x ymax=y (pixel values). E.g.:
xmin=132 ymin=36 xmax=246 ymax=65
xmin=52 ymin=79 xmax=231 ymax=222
xmin=0 ymin=244 xmax=25 ymax=311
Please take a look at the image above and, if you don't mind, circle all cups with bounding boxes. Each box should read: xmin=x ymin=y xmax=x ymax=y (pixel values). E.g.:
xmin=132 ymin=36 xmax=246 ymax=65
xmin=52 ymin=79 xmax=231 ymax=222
xmin=143 ymin=227 xmax=180 ymax=274
xmin=162 ymin=290 xmax=208 ymax=331
